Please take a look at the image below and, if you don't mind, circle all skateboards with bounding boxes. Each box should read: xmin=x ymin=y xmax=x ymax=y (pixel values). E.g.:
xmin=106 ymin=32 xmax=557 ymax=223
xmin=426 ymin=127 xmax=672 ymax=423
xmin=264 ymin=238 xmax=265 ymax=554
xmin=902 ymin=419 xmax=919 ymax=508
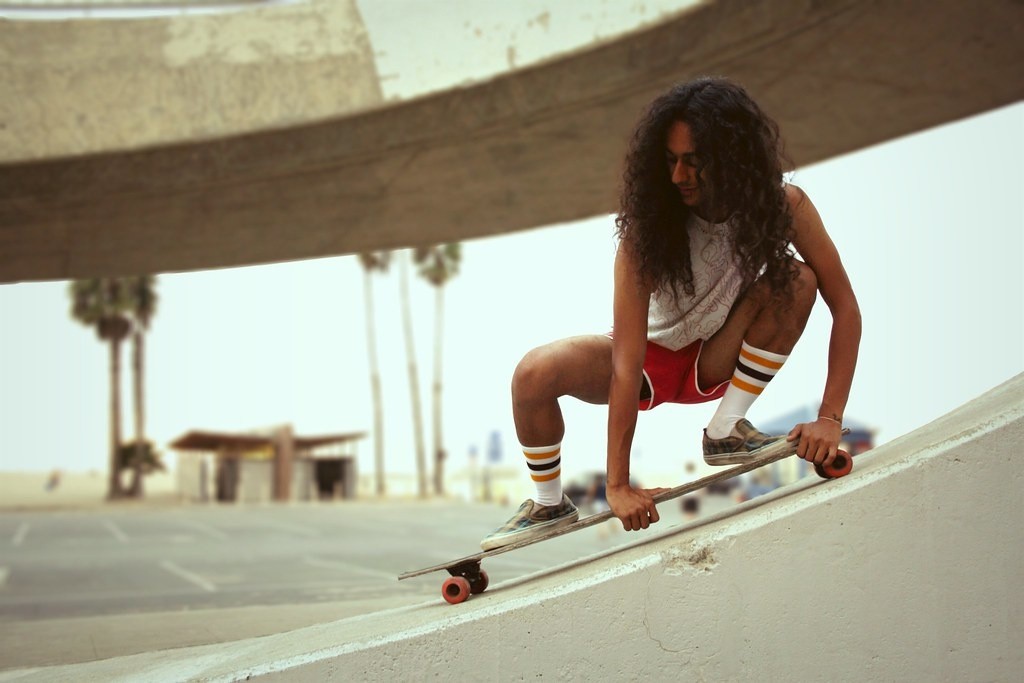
xmin=396 ymin=426 xmax=859 ymax=606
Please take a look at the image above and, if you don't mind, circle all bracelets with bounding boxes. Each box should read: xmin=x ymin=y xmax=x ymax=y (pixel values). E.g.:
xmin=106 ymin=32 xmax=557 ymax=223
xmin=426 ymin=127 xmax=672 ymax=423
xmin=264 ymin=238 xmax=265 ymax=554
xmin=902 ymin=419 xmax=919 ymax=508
xmin=817 ymin=415 xmax=843 ymax=427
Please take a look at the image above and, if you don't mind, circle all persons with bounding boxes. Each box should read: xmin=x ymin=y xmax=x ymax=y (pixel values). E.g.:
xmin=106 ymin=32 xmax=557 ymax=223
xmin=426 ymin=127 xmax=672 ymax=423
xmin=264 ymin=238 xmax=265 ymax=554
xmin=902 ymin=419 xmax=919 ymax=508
xmin=478 ymin=76 xmax=864 ymax=556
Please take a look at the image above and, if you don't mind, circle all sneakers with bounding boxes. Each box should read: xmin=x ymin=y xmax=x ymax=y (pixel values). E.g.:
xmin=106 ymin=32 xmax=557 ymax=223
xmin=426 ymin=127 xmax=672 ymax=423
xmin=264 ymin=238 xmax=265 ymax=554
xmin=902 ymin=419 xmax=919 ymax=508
xmin=703 ymin=418 xmax=787 ymax=466
xmin=480 ymin=492 xmax=579 ymax=552
